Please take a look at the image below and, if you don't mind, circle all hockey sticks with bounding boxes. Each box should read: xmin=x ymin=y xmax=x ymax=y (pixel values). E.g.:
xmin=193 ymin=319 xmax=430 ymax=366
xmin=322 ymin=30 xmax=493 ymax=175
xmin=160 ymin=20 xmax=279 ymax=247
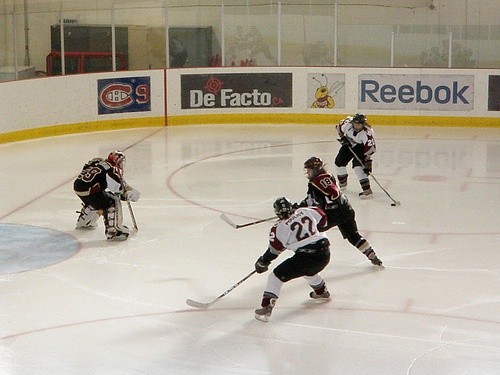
xmin=219 ymin=213 xmax=279 ymax=229
xmin=112 ymin=151 xmax=138 ymax=233
xmin=336 ymin=139 xmax=401 ymax=207
xmin=186 ymin=269 xmax=257 ymax=309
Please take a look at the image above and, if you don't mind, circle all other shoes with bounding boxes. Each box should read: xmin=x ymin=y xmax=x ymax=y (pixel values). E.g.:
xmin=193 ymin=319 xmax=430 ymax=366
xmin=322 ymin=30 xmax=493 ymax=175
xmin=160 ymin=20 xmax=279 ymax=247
xmin=105 ymin=227 xmax=130 ymax=242
xmin=76 ymin=219 xmax=99 ymax=229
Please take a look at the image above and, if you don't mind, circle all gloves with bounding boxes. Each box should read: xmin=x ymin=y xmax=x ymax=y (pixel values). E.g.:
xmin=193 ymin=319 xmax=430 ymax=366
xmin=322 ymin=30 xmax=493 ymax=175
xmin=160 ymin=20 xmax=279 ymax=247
xmin=363 ymin=159 xmax=372 ymax=176
xmin=254 ymin=256 xmax=271 ymax=273
xmin=340 ymin=136 xmax=352 ymax=150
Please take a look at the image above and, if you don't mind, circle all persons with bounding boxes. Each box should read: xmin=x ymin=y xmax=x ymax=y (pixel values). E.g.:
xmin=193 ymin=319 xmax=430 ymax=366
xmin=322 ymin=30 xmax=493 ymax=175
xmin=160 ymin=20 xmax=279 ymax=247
xmin=292 ymin=155 xmax=385 ymax=268
xmin=227 ymin=22 xmax=476 ymax=67
xmin=73 ymin=149 xmax=140 ymax=241
xmin=334 ymin=112 xmax=376 ymax=199
xmin=255 ymin=196 xmax=332 ymax=321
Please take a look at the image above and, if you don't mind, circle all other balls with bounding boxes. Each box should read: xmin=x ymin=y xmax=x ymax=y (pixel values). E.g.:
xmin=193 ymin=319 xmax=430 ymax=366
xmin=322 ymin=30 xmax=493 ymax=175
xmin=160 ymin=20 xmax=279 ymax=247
xmin=391 ymin=203 xmax=396 ymax=206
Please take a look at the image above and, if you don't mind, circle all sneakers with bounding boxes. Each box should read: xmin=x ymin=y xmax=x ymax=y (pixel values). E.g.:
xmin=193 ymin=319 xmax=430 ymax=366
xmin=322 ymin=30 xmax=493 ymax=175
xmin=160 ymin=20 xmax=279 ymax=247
xmin=254 ymin=306 xmax=272 ymax=322
xmin=309 ymin=291 xmax=330 ymax=303
xmin=338 ymin=181 xmax=348 ymax=192
xmin=371 ymin=257 xmax=385 ymax=270
xmin=358 ymin=189 xmax=375 ymax=200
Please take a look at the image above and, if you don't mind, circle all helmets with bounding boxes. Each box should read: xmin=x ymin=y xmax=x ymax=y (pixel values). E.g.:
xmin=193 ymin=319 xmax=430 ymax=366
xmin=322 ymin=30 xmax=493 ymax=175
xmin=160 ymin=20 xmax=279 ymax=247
xmin=304 ymin=156 xmax=324 ymax=179
xmin=108 ymin=150 xmax=127 ymax=171
xmin=352 ymin=113 xmax=367 ymax=130
xmin=273 ymin=196 xmax=293 ymax=220
